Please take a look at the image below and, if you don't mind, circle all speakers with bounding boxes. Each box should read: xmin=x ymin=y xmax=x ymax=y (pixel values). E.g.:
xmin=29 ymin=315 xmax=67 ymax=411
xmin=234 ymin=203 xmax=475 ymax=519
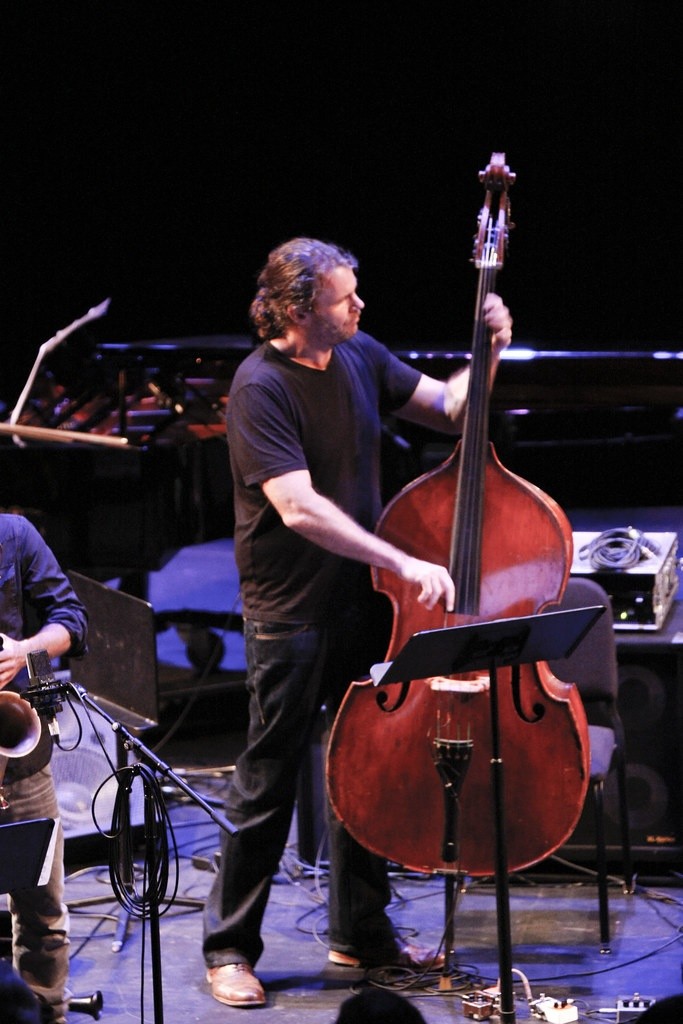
xmin=532 ymin=596 xmax=683 ymax=863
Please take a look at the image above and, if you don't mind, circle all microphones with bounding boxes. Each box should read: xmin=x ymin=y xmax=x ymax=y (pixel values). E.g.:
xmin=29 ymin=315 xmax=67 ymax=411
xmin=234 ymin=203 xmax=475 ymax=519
xmin=27 ymin=649 xmax=61 ymax=745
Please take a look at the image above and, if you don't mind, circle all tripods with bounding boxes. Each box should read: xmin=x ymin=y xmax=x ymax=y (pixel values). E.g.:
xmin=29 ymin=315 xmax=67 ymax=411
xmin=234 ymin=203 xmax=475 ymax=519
xmin=63 ymin=569 xmax=207 ymax=952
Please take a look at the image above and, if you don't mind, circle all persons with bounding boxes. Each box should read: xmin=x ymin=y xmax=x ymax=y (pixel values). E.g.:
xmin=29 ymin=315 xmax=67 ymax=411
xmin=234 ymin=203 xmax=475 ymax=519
xmin=200 ymin=238 xmax=513 ymax=1005
xmin=0 ymin=513 xmax=88 ymax=1024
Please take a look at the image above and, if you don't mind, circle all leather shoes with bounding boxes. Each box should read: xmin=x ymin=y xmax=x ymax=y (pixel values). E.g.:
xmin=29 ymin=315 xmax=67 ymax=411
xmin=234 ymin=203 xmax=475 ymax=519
xmin=205 ymin=954 xmax=269 ymax=1006
xmin=328 ymin=939 xmax=449 ymax=969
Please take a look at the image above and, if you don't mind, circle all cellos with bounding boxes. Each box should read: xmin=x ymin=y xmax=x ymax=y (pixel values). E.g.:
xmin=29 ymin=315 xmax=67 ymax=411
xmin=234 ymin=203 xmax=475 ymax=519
xmin=323 ymin=154 xmax=590 ymax=994
